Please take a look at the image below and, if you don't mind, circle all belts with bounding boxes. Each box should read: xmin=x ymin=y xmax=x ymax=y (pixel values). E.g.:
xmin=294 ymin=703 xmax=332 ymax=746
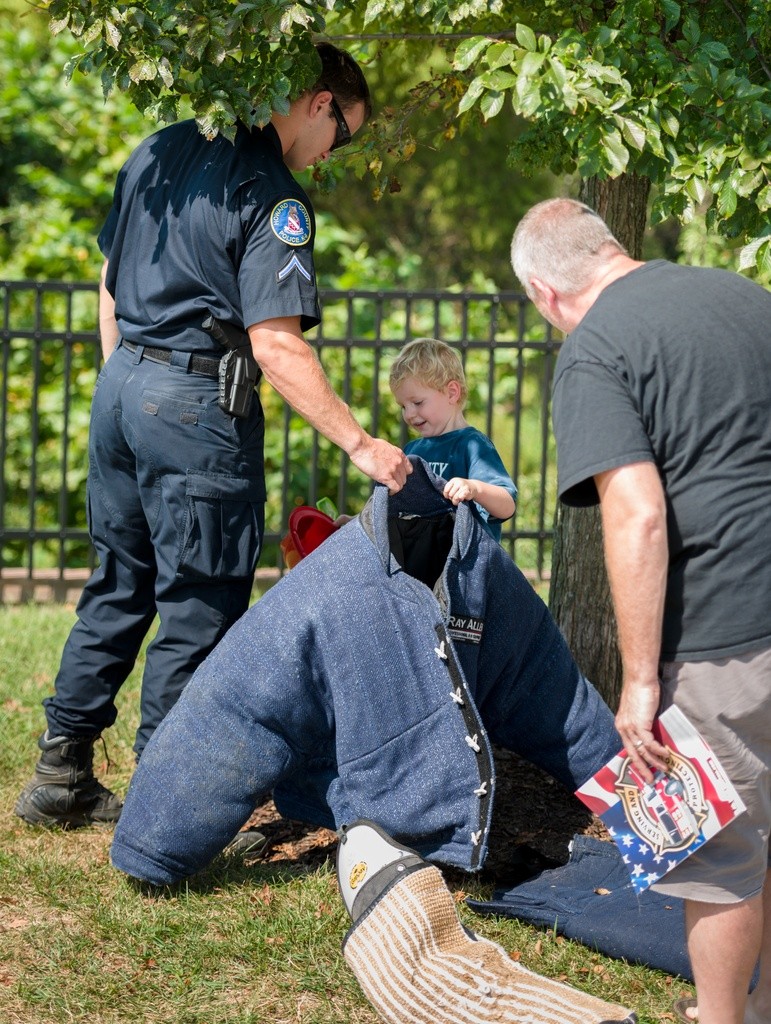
xmin=122 ymin=340 xmax=222 ymax=377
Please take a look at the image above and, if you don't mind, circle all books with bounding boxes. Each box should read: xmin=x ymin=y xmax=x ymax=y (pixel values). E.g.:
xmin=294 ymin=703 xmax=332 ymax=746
xmin=574 ymin=703 xmax=744 ymax=896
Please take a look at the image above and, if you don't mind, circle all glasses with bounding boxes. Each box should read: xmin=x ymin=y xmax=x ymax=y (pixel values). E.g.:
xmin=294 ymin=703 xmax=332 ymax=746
xmin=316 ymin=85 xmax=352 ymax=152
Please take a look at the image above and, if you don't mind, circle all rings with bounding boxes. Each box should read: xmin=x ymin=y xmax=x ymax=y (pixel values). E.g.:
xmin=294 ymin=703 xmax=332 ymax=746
xmin=632 ymin=741 xmax=642 ymax=749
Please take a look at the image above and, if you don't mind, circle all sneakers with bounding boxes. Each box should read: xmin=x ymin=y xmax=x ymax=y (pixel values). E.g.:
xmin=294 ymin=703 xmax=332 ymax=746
xmin=15 ymin=763 xmax=123 ymax=826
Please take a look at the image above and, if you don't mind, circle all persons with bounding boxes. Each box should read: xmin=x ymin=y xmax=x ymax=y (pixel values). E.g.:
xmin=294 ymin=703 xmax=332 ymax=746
xmin=384 ymin=329 xmax=520 ymax=547
xmin=512 ymin=198 xmax=771 ymax=1024
xmin=12 ymin=40 xmax=414 ymax=833
